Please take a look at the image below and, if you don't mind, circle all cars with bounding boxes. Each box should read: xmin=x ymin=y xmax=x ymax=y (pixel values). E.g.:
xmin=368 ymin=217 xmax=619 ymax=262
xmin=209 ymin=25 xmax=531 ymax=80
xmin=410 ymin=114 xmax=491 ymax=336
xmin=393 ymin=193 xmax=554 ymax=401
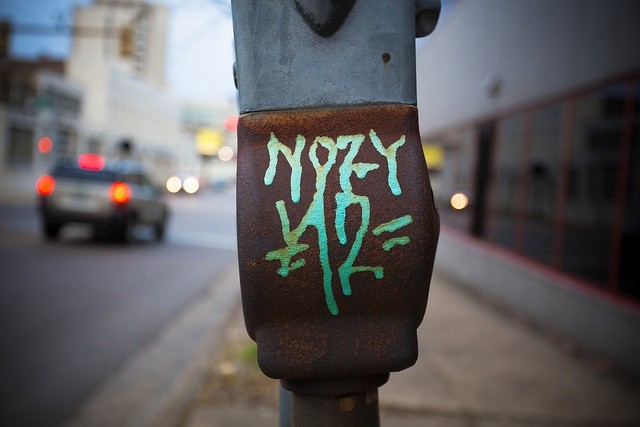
xmin=35 ymin=154 xmax=171 ymax=245
